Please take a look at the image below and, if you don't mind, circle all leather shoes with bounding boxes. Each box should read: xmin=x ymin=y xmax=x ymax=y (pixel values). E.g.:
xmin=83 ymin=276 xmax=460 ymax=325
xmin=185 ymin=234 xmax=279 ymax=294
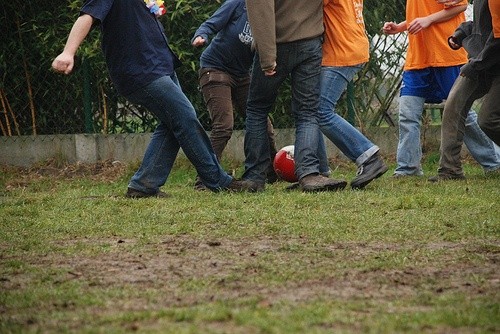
xmin=350 ymin=159 xmax=388 ymax=189
xmin=304 ymin=174 xmax=344 ymax=191
xmin=429 ymin=171 xmax=466 ymax=182
xmin=208 ymin=180 xmax=258 ymax=194
xmin=125 ymin=188 xmax=171 ymax=198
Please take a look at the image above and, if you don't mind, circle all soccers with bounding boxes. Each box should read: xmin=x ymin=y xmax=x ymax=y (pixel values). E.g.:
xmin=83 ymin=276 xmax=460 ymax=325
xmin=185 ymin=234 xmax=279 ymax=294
xmin=273 ymin=144 xmax=299 ymax=183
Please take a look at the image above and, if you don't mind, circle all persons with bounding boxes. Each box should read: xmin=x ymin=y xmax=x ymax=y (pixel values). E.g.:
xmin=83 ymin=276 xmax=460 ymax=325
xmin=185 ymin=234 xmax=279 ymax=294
xmin=286 ymin=0 xmax=388 ymax=189
xmin=383 ymin=0 xmax=500 ymax=178
xmin=190 ymin=0 xmax=281 ymax=192
xmin=427 ymin=0 xmax=500 ymax=182
xmin=52 ymin=0 xmax=259 ymax=199
xmin=242 ymin=0 xmax=348 ymax=193
